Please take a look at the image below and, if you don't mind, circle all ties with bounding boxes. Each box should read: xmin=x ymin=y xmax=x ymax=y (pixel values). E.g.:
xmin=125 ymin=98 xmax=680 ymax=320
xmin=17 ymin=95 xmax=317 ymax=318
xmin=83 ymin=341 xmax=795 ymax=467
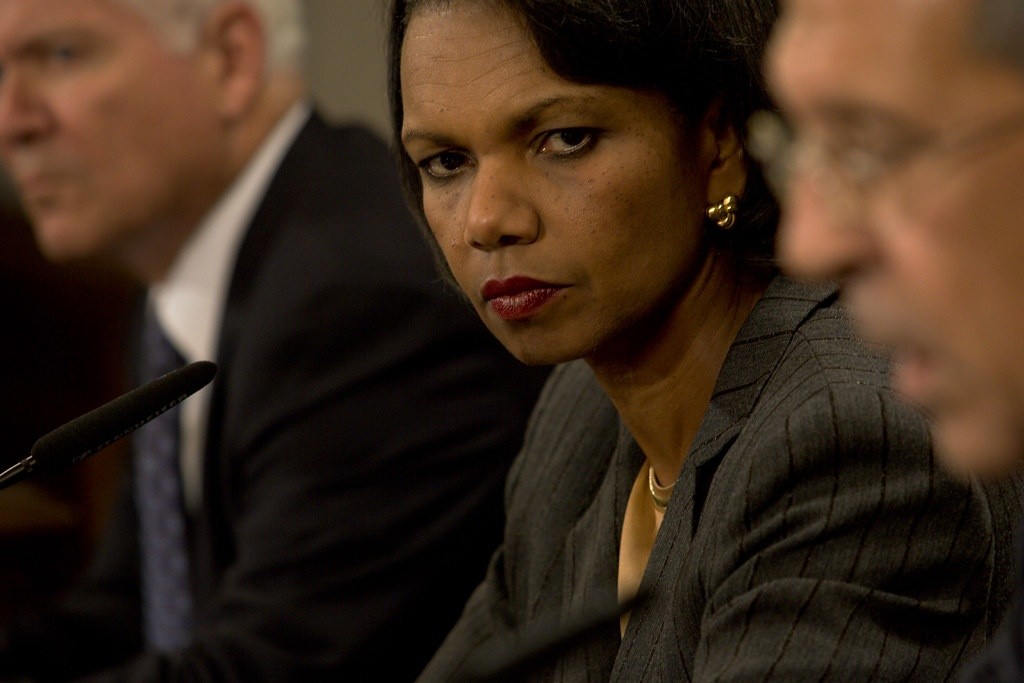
xmin=134 ymin=299 xmax=189 ymax=658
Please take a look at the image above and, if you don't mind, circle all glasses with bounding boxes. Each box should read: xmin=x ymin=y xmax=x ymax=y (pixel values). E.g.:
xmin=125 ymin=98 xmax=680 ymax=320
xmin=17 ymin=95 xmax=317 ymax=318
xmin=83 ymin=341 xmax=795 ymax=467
xmin=742 ymin=97 xmax=1024 ymax=186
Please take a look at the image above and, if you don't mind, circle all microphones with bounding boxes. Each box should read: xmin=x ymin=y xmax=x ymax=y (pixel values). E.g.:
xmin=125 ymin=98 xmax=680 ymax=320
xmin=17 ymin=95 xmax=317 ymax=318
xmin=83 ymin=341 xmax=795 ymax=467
xmin=1 ymin=359 xmax=220 ymax=492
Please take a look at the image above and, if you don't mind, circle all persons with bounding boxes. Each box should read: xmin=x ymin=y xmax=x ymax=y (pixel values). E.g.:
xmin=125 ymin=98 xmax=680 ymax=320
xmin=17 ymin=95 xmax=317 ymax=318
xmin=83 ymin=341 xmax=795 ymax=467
xmin=766 ymin=0 xmax=1024 ymax=683
xmin=388 ymin=0 xmax=1024 ymax=683
xmin=0 ymin=0 xmax=557 ymax=683
